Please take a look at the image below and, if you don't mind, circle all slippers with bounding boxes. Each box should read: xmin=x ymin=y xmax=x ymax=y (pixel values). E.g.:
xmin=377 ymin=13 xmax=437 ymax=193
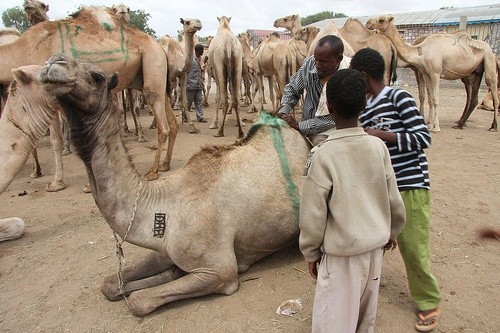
xmin=415 ymin=308 xmax=440 ymax=331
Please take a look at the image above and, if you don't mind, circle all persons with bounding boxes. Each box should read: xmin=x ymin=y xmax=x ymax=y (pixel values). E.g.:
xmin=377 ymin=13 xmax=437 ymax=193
xmin=351 ymin=47 xmax=443 ymax=333
xmin=278 ymin=36 xmax=351 ymax=147
xmin=177 ymin=44 xmax=209 ymax=122
xmin=298 ymin=69 xmax=406 ymax=333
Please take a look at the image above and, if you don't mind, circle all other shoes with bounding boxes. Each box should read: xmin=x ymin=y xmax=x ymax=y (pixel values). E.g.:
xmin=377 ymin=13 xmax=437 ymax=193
xmin=183 ymin=117 xmax=208 ymax=123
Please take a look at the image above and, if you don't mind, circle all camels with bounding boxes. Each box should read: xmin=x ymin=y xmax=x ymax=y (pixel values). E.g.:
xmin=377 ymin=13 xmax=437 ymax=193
xmin=0 ymin=0 xmax=500 ymax=241
xmin=39 ymin=54 xmax=329 ymax=317
